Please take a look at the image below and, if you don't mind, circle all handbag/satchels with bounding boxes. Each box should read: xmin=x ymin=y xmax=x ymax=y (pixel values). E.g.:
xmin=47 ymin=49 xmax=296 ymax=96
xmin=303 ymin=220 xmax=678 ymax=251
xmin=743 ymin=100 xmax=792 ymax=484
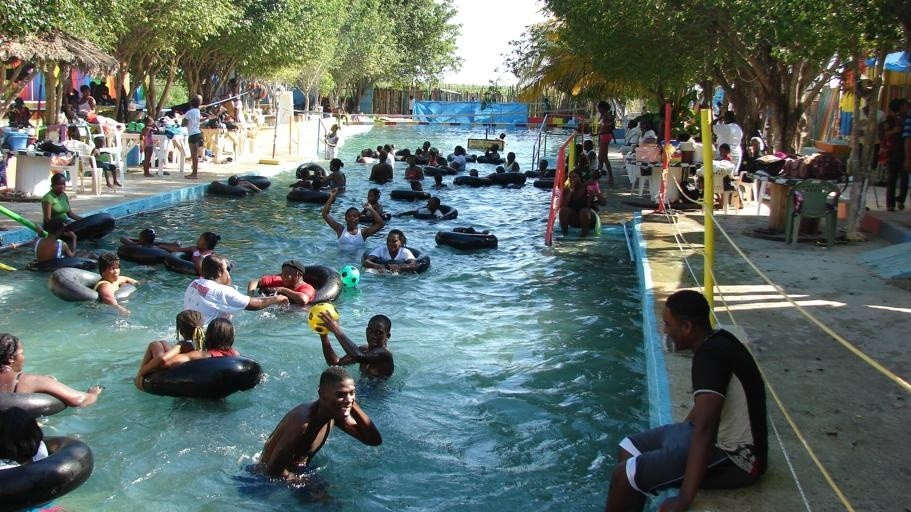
xmin=38 ymin=141 xmax=66 ymax=154
xmin=747 ymin=150 xmax=845 ymax=180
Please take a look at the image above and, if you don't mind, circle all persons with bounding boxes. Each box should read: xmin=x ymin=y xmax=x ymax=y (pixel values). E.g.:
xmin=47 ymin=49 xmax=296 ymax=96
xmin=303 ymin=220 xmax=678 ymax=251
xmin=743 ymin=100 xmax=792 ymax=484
xmin=1 ymin=405 xmax=67 ymax=512
xmin=319 ymin=317 xmax=397 ymax=375
xmin=857 ymin=100 xmax=910 ymax=214
xmin=1 ymin=331 xmax=103 ymax=411
xmin=237 ymin=368 xmax=382 ymax=502
xmin=135 ymin=307 xmax=204 ymax=393
xmin=160 ymin=317 xmax=240 ymax=368
xmin=0 ymin=77 xmax=766 ymax=323
xmin=605 ymin=289 xmax=767 ymax=512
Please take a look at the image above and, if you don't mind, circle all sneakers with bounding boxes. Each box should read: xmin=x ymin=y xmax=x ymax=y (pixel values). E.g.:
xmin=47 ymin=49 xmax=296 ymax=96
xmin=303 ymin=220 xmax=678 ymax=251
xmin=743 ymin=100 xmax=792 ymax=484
xmin=887 ymin=206 xmax=895 ymax=211
xmin=895 ymin=196 xmax=904 ymax=210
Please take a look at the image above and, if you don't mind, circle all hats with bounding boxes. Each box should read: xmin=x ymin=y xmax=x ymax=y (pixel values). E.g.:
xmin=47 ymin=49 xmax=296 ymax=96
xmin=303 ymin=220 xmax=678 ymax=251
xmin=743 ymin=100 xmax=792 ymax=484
xmin=282 ymin=260 xmax=306 ymax=275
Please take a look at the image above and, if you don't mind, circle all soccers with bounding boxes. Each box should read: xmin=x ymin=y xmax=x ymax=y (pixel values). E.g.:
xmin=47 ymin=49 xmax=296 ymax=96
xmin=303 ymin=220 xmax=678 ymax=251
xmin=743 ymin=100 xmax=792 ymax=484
xmin=340 ymin=265 xmax=359 ymax=287
xmin=308 ymin=303 xmax=338 ymax=334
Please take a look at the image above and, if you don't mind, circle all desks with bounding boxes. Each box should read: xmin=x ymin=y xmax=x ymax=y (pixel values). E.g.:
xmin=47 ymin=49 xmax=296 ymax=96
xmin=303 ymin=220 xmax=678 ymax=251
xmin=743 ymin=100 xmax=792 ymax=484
xmin=617 ymin=140 xmax=700 ymax=206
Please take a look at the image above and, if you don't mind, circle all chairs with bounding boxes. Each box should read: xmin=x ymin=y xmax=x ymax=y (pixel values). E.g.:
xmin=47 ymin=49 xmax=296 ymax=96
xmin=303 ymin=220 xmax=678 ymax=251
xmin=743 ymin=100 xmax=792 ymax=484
xmin=698 ymin=163 xmax=873 ymax=250
xmin=1 ymin=96 xmax=311 ymax=203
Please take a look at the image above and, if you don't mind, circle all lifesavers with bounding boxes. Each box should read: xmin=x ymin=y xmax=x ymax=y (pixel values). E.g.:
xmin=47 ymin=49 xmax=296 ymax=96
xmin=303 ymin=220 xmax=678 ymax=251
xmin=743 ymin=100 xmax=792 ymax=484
xmin=50 ymin=267 xmax=137 ymax=301
xmin=414 ymin=204 xmax=458 ymax=221
xmin=62 ymin=213 xmax=114 ymax=239
xmin=287 ymin=189 xmax=337 ymax=204
xmin=362 ymin=247 xmax=430 ymax=275
xmin=258 ymin=265 xmax=341 ymax=305
xmin=210 ymin=181 xmax=254 ymax=197
xmin=142 ymin=354 xmax=260 ymax=395
xmin=407 ymin=156 xmax=458 ymax=175
xmin=390 ymin=190 xmax=432 ymax=202
xmin=1 ymin=391 xmax=67 ymax=419
xmin=477 ymin=155 xmax=506 ymax=164
xmin=118 ymin=244 xmax=179 ymax=263
xmin=238 ymin=176 xmax=271 ymax=189
xmin=534 ymin=179 xmax=554 ymax=188
xmin=0 ymin=437 xmax=93 ymax=511
xmin=296 ymin=162 xmax=329 ymax=181
xmin=526 ymin=169 xmax=556 ymax=177
xmin=357 ymin=149 xmax=380 ymax=164
xmin=488 ymin=173 xmax=526 ymax=185
xmin=164 ymin=251 xmax=233 ymax=275
xmin=447 ymin=153 xmax=477 ymax=163
xmin=359 ymin=211 xmax=391 ymax=222
xmin=453 ymin=175 xmax=492 ymax=187
xmin=435 ymin=231 xmax=498 ymax=251
